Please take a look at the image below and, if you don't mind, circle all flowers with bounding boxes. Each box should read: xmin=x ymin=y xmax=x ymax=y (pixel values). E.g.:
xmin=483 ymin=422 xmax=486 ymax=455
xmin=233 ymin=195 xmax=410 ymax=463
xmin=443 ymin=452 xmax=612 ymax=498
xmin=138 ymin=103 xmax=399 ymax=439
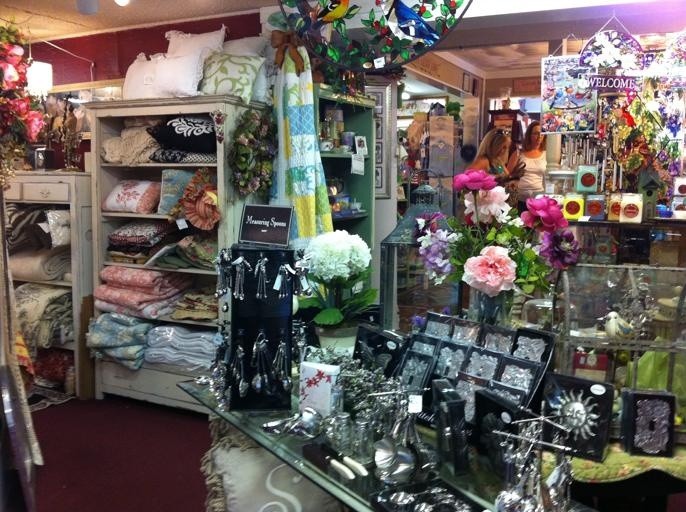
xmin=418 ymin=169 xmax=579 ymax=296
xmin=0 ymin=21 xmax=44 ymax=142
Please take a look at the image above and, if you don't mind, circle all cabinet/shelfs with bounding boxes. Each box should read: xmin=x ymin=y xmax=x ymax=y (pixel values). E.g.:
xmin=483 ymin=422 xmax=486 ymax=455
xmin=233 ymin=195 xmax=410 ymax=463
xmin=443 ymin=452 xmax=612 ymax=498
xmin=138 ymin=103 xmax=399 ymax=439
xmin=0 ymin=172 xmax=94 ymax=403
xmin=316 ymin=81 xmax=379 ymax=306
xmin=84 ymin=93 xmax=270 ymax=416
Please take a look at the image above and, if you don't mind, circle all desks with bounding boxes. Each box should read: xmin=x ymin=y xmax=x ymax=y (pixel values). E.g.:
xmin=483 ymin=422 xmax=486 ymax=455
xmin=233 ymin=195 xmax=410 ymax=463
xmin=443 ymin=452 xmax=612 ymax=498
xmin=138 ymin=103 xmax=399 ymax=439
xmin=178 ymin=373 xmax=510 ymax=512
xmin=379 ymin=203 xmax=452 ymax=331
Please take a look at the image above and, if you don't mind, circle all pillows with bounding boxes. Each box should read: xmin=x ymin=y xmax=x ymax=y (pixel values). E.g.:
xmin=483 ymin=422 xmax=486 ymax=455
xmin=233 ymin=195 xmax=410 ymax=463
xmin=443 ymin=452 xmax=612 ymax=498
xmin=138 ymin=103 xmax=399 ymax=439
xmin=121 ymin=25 xmax=273 ymax=105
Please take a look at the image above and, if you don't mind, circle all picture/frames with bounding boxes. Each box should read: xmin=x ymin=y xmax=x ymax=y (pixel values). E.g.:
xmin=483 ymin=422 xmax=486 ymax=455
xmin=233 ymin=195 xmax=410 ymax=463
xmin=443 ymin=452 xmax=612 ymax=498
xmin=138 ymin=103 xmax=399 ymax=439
xmin=363 ymin=80 xmax=392 ymax=199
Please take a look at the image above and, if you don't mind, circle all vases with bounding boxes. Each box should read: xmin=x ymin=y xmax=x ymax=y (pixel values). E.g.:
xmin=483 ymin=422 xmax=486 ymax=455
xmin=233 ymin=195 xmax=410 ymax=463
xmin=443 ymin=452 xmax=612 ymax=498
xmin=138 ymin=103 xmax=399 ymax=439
xmin=296 ymin=229 xmax=377 ymax=361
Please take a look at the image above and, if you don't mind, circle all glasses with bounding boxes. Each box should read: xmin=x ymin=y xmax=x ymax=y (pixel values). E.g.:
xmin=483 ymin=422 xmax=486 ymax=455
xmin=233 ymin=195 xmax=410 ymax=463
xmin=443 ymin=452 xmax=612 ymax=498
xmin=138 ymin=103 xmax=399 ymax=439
xmin=494 ymin=129 xmax=511 ymax=137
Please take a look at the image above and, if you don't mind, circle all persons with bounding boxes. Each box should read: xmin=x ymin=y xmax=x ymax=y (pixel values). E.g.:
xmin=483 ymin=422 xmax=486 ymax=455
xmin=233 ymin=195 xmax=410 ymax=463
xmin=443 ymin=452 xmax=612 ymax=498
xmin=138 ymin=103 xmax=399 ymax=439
xmin=506 ymin=121 xmax=548 ymax=217
xmin=470 ymin=128 xmax=518 ymax=211
